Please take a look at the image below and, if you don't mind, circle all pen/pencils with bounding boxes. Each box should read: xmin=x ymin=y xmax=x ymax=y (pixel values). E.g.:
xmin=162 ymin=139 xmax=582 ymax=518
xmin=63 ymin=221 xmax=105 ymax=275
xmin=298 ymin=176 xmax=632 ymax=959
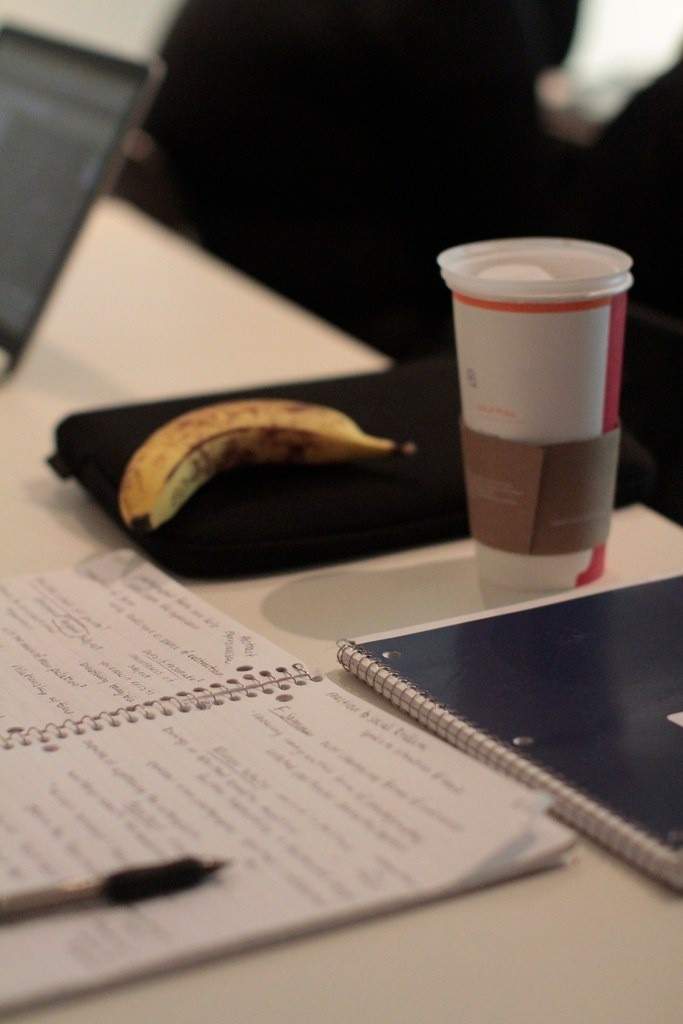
xmin=1 ymin=857 xmax=233 ymax=932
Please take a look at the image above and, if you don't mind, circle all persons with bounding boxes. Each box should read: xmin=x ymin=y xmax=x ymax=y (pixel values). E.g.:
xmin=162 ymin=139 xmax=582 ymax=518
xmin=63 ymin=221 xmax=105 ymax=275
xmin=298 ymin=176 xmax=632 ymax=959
xmin=115 ymin=0 xmax=683 ymax=528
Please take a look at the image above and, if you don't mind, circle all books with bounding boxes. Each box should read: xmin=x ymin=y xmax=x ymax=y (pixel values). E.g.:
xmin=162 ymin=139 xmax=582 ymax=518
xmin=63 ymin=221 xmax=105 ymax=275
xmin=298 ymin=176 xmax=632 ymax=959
xmin=0 ymin=546 xmax=577 ymax=1016
xmin=330 ymin=572 xmax=683 ymax=893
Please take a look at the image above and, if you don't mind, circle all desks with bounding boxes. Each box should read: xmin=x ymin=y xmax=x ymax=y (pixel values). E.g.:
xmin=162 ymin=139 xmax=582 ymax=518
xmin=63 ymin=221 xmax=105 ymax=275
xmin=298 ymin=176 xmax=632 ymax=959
xmin=0 ymin=194 xmax=683 ymax=1024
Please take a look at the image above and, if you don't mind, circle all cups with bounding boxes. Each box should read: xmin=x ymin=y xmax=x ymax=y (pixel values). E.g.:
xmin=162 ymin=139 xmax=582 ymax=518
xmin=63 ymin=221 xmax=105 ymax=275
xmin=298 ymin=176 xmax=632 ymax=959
xmin=437 ymin=235 xmax=635 ymax=594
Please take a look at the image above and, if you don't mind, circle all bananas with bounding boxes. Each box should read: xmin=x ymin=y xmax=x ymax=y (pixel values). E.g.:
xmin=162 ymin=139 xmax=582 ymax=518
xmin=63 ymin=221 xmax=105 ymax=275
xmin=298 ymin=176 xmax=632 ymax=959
xmin=117 ymin=399 xmax=418 ymax=537
